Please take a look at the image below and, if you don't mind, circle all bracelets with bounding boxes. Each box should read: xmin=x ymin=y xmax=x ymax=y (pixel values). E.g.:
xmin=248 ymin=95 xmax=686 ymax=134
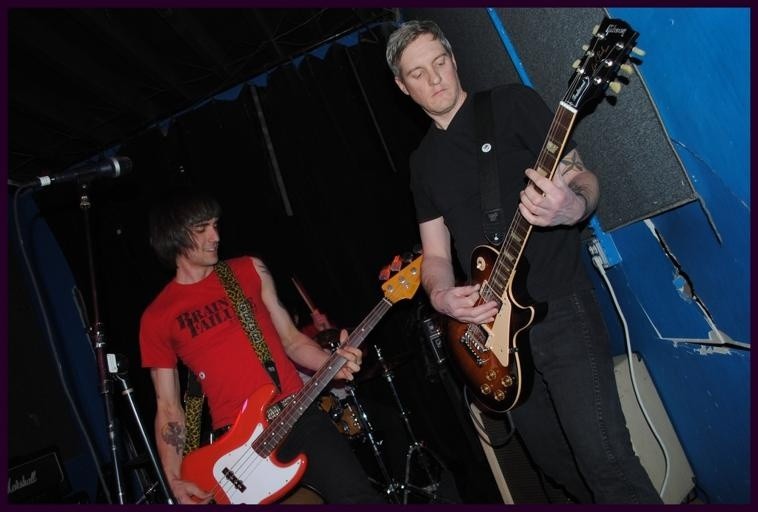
xmin=573 ymin=191 xmax=587 ymax=226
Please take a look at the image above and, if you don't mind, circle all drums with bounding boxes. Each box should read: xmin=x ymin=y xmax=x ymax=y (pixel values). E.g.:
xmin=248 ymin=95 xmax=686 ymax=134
xmin=316 ymin=396 xmax=367 ymax=444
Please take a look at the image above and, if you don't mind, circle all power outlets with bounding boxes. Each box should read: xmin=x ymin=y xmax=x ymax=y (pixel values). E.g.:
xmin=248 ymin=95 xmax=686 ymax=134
xmin=585 ymin=237 xmax=607 ymax=269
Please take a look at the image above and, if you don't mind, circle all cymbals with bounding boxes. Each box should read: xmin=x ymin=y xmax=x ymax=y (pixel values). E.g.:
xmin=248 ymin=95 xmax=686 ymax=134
xmin=312 ymin=329 xmax=385 ymax=368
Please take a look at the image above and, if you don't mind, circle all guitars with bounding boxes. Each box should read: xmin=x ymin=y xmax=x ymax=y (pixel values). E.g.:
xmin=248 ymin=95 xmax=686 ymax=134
xmin=182 ymin=243 xmax=423 ymax=506
xmin=445 ymin=15 xmax=646 ymax=415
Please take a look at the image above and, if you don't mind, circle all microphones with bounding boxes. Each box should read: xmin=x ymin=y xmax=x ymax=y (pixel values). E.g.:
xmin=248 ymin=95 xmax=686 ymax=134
xmin=37 ymin=154 xmax=132 ymax=190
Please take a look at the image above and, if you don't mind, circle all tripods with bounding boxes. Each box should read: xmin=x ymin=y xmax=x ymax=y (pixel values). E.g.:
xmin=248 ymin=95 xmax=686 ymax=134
xmin=374 ymin=347 xmax=460 ymax=503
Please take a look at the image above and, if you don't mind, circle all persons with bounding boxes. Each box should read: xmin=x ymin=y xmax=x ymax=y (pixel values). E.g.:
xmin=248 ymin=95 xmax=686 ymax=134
xmin=310 ymin=309 xmax=331 ymax=331
xmin=135 ymin=192 xmax=388 ymax=504
xmin=385 ymin=18 xmax=665 ymax=505
